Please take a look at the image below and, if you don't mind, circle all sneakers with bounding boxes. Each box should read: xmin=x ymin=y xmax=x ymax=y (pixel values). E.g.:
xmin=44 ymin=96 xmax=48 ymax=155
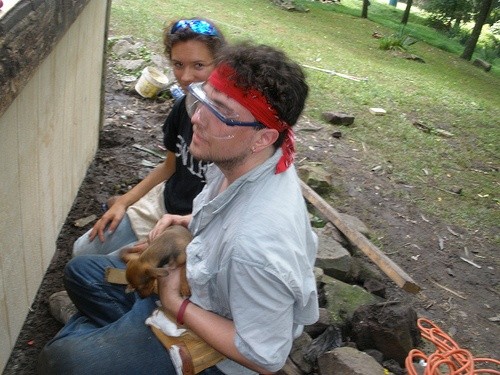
xmin=49 ymin=291 xmax=79 ymax=325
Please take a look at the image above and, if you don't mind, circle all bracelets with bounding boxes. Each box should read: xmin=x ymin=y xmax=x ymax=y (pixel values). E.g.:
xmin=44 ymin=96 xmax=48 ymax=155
xmin=177 ymin=298 xmax=191 ymax=325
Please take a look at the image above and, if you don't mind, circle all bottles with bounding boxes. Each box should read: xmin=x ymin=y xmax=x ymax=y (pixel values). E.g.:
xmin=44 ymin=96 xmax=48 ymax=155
xmin=170 ymin=85 xmax=184 ymax=102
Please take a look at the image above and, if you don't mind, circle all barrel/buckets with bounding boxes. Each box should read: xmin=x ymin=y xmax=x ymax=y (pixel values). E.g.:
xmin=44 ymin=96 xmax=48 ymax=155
xmin=135 ymin=66 xmax=169 ymax=99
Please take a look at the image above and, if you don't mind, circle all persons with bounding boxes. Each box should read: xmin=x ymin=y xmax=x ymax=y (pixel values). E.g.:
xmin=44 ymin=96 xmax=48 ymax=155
xmin=40 ymin=40 xmax=320 ymax=375
xmin=73 ymin=17 xmax=229 ymax=256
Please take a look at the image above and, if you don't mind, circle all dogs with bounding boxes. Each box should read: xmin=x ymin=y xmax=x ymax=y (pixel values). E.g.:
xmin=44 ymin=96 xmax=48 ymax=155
xmin=121 ymin=224 xmax=193 ymax=298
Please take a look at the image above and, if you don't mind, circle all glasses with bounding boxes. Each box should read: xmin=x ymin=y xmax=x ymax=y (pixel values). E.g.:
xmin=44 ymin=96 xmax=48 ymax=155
xmin=185 ymin=81 xmax=266 ymax=139
xmin=170 ymin=19 xmax=221 ymax=38
